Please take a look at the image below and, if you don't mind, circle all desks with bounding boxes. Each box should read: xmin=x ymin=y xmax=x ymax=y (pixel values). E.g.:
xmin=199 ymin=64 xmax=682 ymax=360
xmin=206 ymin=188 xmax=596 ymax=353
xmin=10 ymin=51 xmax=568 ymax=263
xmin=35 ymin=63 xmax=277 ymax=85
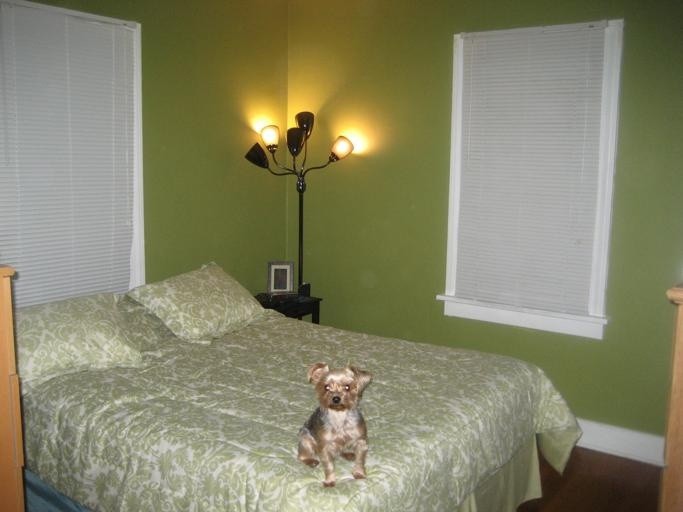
xmin=658 ymin=284 xmax=683 ymax=510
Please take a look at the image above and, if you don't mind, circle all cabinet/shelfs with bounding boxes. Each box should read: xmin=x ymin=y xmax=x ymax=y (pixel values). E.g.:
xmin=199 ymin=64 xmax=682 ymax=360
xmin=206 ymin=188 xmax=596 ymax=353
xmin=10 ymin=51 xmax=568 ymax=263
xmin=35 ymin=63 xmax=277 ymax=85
xmin=0 ymin=266 xmax=29 ymax=510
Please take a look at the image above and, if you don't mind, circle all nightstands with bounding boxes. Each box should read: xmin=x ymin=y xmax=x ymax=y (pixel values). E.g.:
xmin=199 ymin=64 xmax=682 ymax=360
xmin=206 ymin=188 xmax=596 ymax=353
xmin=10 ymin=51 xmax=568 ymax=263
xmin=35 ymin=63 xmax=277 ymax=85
xmin=251 ymin=293 xmax=323 ymax=325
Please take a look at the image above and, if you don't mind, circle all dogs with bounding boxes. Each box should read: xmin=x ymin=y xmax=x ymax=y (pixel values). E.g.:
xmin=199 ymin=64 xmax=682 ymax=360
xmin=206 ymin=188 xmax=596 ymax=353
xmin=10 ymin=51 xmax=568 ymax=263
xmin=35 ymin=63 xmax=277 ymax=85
xmin=298 ymin=360 xmax=373 ymax=487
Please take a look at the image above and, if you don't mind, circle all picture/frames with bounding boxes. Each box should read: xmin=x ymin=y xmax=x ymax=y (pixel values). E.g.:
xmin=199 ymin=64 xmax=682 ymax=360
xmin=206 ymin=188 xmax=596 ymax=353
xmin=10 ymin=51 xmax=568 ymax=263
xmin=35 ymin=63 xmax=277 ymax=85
xmin=268 ymin=260 xmax=295 ymax=297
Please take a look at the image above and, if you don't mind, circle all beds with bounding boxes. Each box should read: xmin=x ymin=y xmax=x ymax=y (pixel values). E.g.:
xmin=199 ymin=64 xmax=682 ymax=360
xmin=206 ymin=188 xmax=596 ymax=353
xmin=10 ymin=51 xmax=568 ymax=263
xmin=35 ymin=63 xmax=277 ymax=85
xmin=13 ymin=257 xmax=584 ymax=511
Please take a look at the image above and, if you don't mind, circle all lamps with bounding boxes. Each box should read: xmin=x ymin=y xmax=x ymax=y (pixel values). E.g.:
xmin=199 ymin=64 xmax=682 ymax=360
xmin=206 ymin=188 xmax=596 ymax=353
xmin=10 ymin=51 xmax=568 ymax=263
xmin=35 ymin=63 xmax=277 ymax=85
xmin=243 ymin=107 xmax=354 ymax=297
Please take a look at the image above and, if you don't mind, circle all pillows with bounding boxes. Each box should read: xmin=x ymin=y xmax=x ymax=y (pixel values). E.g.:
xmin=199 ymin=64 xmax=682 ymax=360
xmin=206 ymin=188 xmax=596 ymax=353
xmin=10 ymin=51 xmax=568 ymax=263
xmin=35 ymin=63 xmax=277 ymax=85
xmin=124 ymin=262 xmax=261 ymax=348
xmin=16 ymin=285 xmax=145 ymax=395
